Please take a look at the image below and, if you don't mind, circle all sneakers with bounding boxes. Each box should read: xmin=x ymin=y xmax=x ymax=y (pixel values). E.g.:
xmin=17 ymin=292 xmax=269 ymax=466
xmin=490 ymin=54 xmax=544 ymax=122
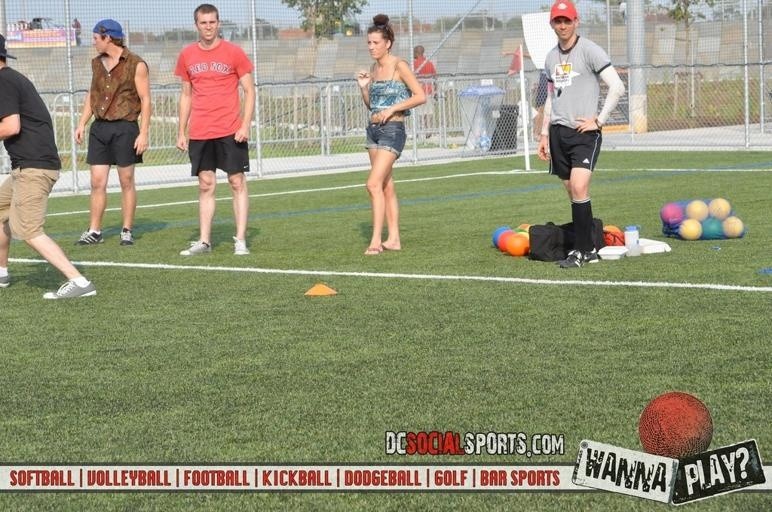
xmin=74 ymin=227 xmax=104 ymax=245
xmin=561 ymin=248 xmax=599 ymax=267
xmin=43 ymin=278 xmax=96 ymax=299
xmin=0 ymin=273 xmax=10 ymax=288
xmin=180 ymin=241 xmax=212 ymax=256
xmin=119 ymin=227 xmax=133 ymax=246
xmin=232 ymin=235 xmax=249 ymax=255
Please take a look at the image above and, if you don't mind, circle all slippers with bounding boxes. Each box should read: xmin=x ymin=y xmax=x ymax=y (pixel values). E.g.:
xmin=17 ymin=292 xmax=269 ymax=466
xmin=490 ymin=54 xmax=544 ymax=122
xmin=363 ymin=240 xmax=390 ymax=255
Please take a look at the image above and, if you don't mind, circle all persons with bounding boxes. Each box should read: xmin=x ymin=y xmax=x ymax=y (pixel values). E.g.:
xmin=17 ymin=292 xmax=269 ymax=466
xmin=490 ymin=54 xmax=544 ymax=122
xmin=532 ymin=0 xmax=627 ymax=269
xmin=71 ymin=16 xmax=150 ymax=251
xmin=1 ymin=34 xmax=98 ymax=304
xmin=413 ymin=45 xmax=440 ymax=144
xmin=173 ymin=3 xmax=257 ymax=260
xmin=355 ymin=14 xmax=429 ymax=259
xmin=70 ymin=18 xmax=82 ymax=46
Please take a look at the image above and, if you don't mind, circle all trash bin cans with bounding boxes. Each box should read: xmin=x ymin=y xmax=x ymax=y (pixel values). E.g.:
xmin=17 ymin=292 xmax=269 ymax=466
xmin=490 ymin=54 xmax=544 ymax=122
xmin=458 ymin=85 xmax=519 ymax=156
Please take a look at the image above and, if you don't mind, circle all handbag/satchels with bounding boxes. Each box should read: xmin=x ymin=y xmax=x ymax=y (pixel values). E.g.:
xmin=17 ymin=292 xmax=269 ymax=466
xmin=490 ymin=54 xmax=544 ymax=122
xmin=528 ymin=219 xmax=605 ymax=259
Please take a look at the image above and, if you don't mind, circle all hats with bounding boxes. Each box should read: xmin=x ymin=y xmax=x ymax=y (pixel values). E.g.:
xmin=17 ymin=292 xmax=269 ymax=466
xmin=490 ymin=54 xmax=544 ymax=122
xmin=94 ymin=19 xmax=126 ymax=39
xmin=0 ymin=34 xmax=17 ymax=59
xmin=550 ymin=0 xmax=578 ymax=21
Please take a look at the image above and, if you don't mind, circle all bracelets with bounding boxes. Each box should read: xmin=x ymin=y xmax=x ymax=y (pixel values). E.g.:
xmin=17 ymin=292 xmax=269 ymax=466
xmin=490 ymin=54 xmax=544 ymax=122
xmin=595 ymin=118 xmax=602 ymax=130
